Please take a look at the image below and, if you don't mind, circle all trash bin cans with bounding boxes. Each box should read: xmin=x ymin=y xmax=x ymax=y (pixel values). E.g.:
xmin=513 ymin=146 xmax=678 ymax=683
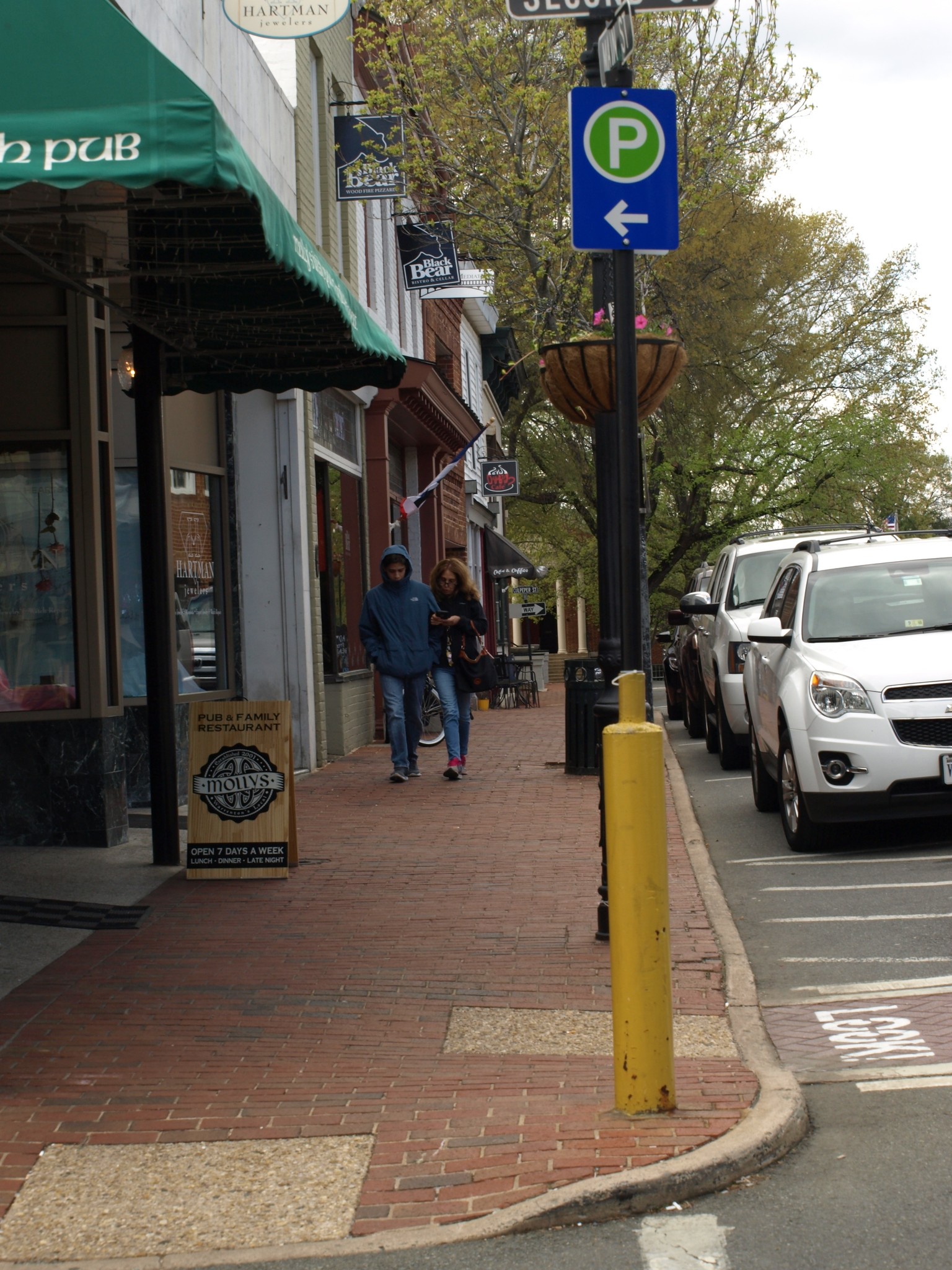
xmin=564 ymin=657 xmax=606 ymax=778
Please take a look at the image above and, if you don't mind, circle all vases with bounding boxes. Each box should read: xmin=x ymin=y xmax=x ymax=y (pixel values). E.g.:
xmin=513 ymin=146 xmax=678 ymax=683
xmin=541 ymin=339 xmax=688 ymax=427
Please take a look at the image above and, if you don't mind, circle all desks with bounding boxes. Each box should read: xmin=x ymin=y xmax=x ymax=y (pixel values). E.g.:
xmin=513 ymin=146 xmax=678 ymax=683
xmin=504 ymin=660 xmax=533 ymax=708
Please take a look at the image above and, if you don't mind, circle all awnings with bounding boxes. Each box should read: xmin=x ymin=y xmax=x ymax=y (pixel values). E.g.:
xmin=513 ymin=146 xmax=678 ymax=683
xmin=0 ymin=1 xmax=409 ymax=398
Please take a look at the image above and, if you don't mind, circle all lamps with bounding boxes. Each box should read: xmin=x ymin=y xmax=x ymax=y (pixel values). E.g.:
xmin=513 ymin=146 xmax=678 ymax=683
xmin=118 ymin=339 xmax=135 ymax=392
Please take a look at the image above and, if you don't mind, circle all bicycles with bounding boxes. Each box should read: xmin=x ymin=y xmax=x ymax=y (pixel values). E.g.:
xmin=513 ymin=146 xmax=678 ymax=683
xmin=414 ymin=673 xmax=449 ymax=746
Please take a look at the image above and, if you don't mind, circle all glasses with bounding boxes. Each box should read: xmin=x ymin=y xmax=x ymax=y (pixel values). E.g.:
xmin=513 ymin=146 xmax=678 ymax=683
xmin=436 ymin=577 xmax=457 ymax=589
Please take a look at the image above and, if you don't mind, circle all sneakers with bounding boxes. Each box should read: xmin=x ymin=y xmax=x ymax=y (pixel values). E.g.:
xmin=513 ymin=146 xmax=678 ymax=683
xmin=407 ymin=765 xmax=422 ymax=776
xmin=389 ymin=766 xmax=408 ymax=781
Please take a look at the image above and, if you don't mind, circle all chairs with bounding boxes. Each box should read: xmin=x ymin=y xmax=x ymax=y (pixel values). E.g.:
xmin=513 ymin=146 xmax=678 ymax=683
xmin=493 ymin=652 xmax=540 ymax=709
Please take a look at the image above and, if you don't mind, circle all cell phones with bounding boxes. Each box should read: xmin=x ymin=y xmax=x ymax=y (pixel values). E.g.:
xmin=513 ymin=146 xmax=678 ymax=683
xmin=436 ymin=610 xmax=450 ymax=620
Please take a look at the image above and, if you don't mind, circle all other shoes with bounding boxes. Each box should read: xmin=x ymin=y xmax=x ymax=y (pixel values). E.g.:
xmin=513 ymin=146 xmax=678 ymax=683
xmin=443 ymin=757 xmax=462 ymax=780
xmin=460 ymin=755 xmax=467 ymax=774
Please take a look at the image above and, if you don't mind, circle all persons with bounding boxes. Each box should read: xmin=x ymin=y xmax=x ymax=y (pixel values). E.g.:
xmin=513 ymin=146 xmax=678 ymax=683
xmin=427 ymin=558 xmax=489 ymax=779
xmin=357 ymin=544 xmax=447 ymax=781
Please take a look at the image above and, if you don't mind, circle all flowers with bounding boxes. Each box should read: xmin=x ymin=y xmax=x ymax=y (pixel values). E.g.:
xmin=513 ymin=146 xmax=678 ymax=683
xmin=30 ymin=493 xmax=52 ymax=592
xmin=40 ymin=471 xmax=64 ymax=554
xmin=499 ymin=309 xmax=674 ymax=380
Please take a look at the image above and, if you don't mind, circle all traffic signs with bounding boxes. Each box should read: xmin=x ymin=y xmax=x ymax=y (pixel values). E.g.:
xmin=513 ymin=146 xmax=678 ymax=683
xmin=572 ymin=86 xmax=680 ymax=251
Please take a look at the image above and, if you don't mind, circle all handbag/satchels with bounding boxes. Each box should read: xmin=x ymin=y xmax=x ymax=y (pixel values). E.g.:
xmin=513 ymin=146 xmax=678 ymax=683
xmin=453 ymin=619 xmax=499 ymax=693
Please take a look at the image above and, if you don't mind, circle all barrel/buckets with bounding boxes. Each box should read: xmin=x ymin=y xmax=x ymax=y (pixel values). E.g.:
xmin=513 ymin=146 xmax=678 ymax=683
xmin=479 ymin=699 xmax=489 ymax=710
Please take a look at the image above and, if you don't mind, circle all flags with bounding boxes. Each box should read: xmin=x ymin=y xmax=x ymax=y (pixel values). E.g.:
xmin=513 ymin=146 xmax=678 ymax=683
xmin=399 ymin=427 xmax=488 ymax=519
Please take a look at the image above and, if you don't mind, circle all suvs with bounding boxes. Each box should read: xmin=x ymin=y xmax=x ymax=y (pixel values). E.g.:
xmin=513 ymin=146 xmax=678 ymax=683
xmin=654 ymin=560 xmax=717 ymax=739
xmin=680 ymin=521 xmax=904 ymax=773
xmin=173 ymin=584 xmax=219 ymax=690
xmin=742 ymin=527 xmax=952 ymax=854
